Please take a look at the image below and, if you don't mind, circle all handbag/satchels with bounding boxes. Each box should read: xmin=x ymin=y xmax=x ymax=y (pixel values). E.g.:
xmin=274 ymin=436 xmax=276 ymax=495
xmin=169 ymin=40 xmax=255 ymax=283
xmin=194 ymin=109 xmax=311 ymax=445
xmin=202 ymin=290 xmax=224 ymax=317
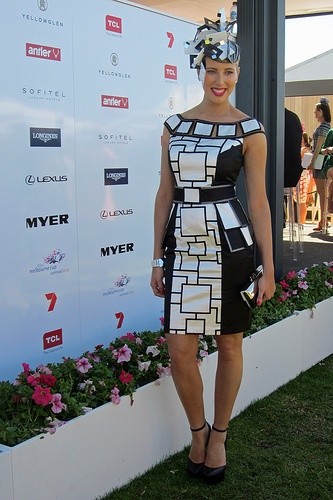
xmin=300 ymin=152 xmax=324 ymax=168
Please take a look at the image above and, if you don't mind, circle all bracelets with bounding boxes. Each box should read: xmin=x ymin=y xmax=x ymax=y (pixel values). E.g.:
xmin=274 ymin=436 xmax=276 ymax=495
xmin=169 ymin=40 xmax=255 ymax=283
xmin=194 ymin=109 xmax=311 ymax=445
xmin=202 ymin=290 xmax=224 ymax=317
xmin=151 ymin=258 xmax=165 ymax=268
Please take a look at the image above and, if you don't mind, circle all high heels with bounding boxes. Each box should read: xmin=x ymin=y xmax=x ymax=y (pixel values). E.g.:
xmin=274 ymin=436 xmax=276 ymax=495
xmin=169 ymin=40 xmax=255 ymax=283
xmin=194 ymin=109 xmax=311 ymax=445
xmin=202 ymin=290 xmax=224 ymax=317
xmin=188 ymin=420 xmax=229 ymax=483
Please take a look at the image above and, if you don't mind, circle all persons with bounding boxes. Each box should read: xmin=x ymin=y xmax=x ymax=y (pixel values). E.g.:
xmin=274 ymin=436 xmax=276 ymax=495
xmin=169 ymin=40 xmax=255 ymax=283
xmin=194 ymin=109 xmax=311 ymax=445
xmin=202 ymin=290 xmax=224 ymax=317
xmin=149 ymin=5 xmax=277 ymax=484
xmin=283 ymin=98 xmax=333 ymax=231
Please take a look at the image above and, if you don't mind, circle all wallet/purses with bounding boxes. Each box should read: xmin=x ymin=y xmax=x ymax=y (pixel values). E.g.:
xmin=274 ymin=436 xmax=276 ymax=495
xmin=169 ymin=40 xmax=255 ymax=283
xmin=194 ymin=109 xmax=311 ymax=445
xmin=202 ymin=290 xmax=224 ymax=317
xmin=240 ymin=264 xmax=264 ymax=309
